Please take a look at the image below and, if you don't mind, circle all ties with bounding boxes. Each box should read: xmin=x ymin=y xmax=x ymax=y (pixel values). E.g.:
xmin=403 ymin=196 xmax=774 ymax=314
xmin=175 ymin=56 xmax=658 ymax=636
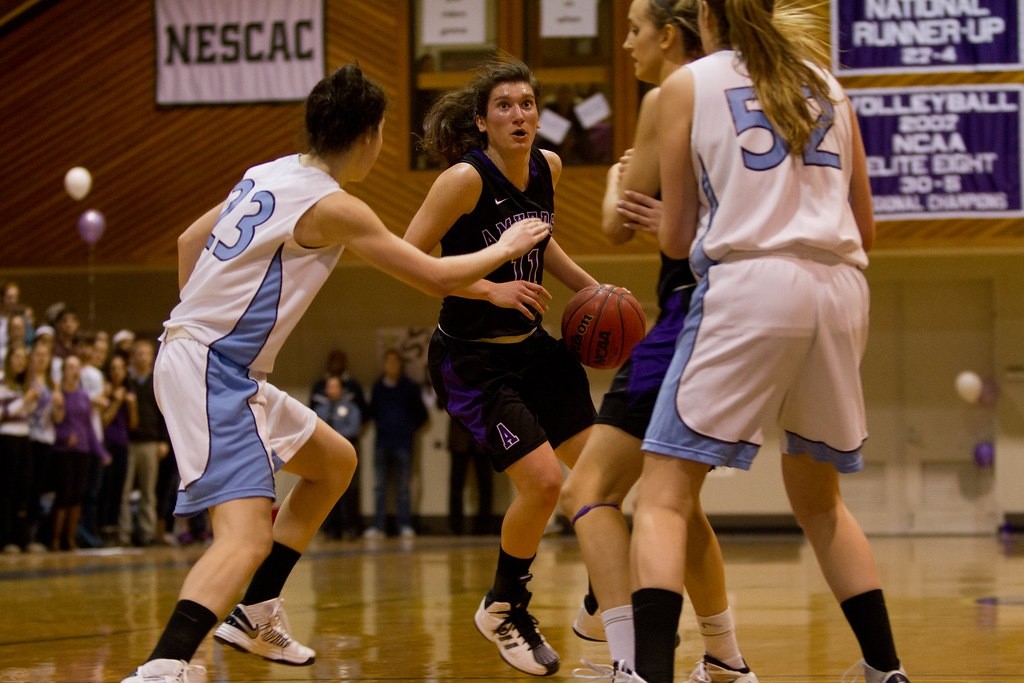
xmin=327 ymin=402 xmax=335 ymax=427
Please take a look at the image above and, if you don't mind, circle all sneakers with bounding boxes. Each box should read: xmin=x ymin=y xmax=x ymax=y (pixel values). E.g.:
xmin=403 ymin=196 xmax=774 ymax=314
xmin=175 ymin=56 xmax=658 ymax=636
xmin=120 ymin=658 xmax=207 ymax=683
xmin=840 ymin=653 xmax=910 ymax=683
xmin=213 ymin=596 xmax=317 ymax=667
xmin=474 ymin=586 xmax=562 ymax=677
xmin=572 ymin=594 xmax=681 ymax=648
xmin=684 ymin=653 xmax=759 ymax=683
xmin=570 ymin=658 xmax=650 ymax=683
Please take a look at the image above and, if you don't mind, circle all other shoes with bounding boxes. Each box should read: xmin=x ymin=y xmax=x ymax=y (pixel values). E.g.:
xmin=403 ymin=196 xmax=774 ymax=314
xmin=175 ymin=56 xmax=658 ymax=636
xmin=401 ymin=527 xmax=416 ymax=539
xmin=28 ymin=542 xmax=47 ymax=553
xmin=365 ymin=527 xmax=389 ymax=541
xmin=5 ymin=544 xmax=21 ymax=555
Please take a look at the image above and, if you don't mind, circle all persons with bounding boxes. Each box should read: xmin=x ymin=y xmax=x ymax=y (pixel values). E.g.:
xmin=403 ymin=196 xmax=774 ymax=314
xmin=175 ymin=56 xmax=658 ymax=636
xmin=1 ymin=282 xmax=169 ymax=554
xmin=556 ymin=0 xmax=913 ymax=683
xmin=117 ymin=62 xmax=642 ymax=683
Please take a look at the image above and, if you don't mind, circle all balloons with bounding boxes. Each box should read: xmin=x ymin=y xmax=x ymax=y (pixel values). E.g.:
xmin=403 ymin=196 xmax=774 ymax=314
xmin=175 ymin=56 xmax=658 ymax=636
xmin=63 ymin=165 xmax=93 ymax=203
xmin=77 ymin=208 xmax=107 ymax=247
xmin=973 ymin=440 xmax=995 ymax=469
xmin=954 ymin=371 xmax=1001 ymax=408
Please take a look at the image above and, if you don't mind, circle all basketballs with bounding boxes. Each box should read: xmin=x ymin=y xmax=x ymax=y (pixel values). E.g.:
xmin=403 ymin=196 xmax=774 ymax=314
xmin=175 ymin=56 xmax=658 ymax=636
xmin=559 ymin=282 xmax=649 ymax=372
